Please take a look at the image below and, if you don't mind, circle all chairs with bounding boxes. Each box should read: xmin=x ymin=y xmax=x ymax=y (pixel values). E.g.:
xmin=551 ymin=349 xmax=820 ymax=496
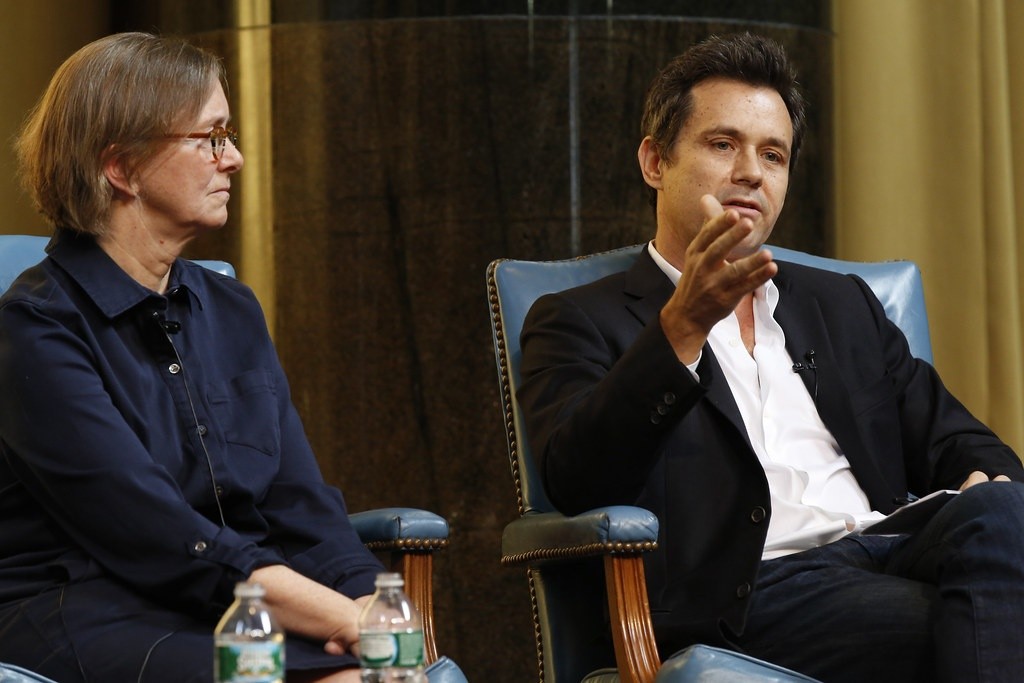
xmin=0 ymin=232 xmax=448 ymax=683
xmin=486 ymin=239 xmax=933 ymax=683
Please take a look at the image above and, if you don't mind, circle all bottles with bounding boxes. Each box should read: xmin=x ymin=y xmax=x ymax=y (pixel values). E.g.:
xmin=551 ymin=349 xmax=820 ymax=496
xmin=359 ymin=571 xmax=424 ymax=683
xmin=212 ymin=582 xmax=286 ymax=683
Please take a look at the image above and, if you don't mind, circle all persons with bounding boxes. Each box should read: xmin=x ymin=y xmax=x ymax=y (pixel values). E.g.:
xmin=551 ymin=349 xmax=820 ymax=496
xmin=0 ymin=32 xmax=427 ymax=683
xmin=514 ymin=29 xmax=1024 ymax=683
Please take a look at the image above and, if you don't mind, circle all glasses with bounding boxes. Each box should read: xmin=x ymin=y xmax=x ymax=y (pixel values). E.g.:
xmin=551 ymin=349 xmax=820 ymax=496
xmin=150 ymin=126 xmax=239 ymax=162
